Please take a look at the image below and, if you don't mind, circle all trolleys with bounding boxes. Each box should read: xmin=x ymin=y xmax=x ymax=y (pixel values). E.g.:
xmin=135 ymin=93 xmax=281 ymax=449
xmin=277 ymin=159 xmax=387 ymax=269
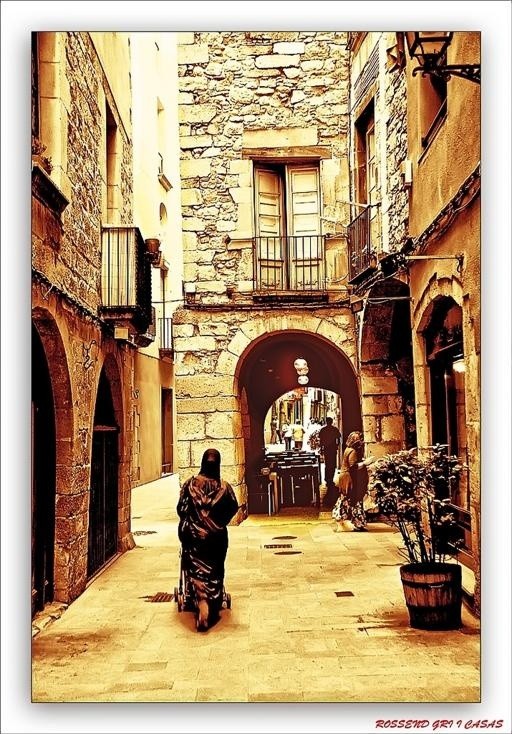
xmin=173 ymin=540 xmax=231 ymax=613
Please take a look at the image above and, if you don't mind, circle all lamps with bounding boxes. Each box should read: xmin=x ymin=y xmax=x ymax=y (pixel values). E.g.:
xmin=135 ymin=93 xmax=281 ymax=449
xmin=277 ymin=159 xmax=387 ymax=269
xmin=405 ymin=32 xmax=480 ymax=86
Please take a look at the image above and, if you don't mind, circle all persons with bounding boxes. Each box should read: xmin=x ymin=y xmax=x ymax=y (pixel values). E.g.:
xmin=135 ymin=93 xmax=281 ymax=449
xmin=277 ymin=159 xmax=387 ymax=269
xmin=318 ymin=416 xmax=342 ymax=487
xmin=174 ymin=447 xmax=239 ymax=631
xmin=331 ymin=429 xmax=377 ymax=533
xmin=268 ymin=413 xmax=326 ymax=463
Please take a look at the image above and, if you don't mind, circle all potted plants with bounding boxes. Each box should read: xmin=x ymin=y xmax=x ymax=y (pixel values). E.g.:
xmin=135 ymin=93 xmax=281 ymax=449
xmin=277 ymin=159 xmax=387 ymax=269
xmin=369 ymin=446 xmax=468 ymax=631
